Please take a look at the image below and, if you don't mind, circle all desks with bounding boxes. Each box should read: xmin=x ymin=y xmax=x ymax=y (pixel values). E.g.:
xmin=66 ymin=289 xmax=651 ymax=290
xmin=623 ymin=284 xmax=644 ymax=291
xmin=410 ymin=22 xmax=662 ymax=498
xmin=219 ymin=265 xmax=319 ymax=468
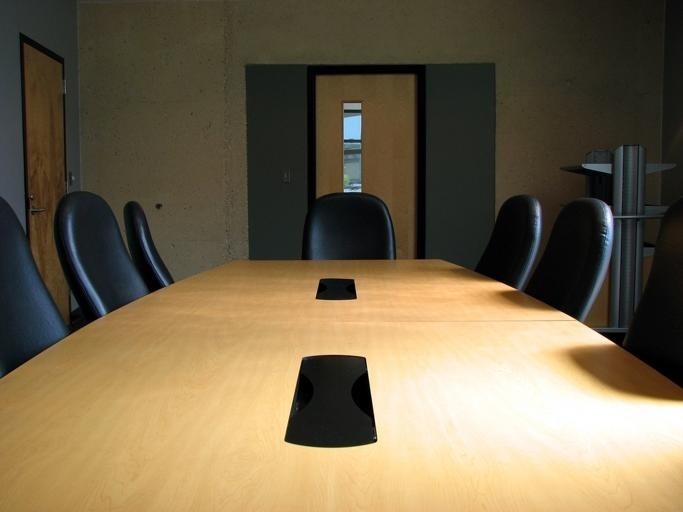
xmin=0 ymin=260 xmax=682 ymax=512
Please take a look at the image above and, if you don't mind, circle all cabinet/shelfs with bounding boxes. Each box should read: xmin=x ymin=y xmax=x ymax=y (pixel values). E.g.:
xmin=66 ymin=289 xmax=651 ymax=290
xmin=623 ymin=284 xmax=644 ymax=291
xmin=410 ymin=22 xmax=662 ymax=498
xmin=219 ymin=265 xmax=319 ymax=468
xmin=560 ymin=145 xmax=677 ymax=333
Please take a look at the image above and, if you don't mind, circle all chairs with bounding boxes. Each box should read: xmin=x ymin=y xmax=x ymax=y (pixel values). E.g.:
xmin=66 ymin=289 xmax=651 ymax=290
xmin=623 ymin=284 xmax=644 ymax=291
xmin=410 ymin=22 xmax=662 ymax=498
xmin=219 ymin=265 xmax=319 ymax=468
xmin=475 ymin=194 xmax=683 ymax=386
xmin=303 ymin=192 xmax=397 ymax=259
xmin=0 ymin=191 xmax=174 ymax=377
